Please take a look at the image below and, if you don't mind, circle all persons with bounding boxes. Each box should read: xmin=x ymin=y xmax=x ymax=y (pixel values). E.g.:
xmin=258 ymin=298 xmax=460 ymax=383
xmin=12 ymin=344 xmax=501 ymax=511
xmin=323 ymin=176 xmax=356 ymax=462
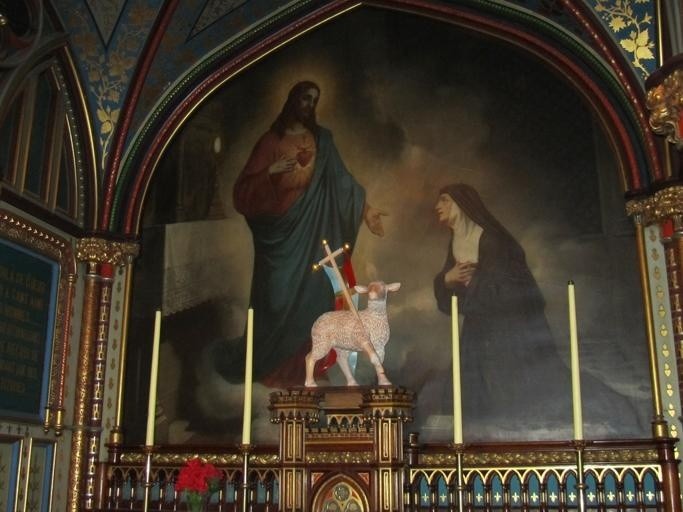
xmin=229 ymin=79 xmax=391 ymax=389
xmin=430 ymin=182 xmax=640 ymax=434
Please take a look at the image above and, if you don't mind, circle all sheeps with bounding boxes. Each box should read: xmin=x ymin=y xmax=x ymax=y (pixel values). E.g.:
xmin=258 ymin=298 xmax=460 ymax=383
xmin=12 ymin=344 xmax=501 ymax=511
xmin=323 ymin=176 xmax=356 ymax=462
xmin=304 ymin=280 xmax=401 ymax=388
